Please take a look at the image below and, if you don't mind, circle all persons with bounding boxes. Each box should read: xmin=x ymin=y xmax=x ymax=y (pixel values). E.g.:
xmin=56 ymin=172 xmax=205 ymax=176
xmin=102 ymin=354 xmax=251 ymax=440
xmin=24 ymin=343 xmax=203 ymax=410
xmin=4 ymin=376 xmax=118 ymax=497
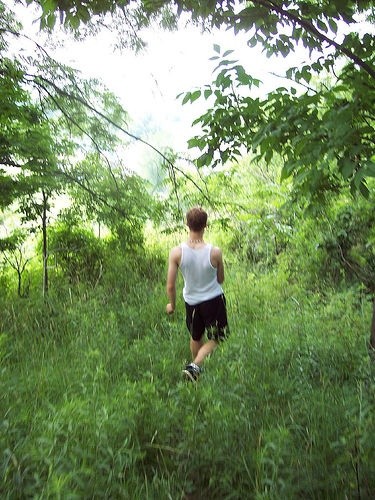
xmin=164 ymin=206 xmax=230 ymax=384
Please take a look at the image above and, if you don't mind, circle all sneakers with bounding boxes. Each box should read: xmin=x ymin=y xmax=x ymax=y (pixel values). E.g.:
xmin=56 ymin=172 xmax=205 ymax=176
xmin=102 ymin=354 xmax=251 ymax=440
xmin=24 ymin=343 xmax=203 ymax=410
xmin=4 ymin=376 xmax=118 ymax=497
xmin=182 ymin=365 xmax=199 ymax=383
